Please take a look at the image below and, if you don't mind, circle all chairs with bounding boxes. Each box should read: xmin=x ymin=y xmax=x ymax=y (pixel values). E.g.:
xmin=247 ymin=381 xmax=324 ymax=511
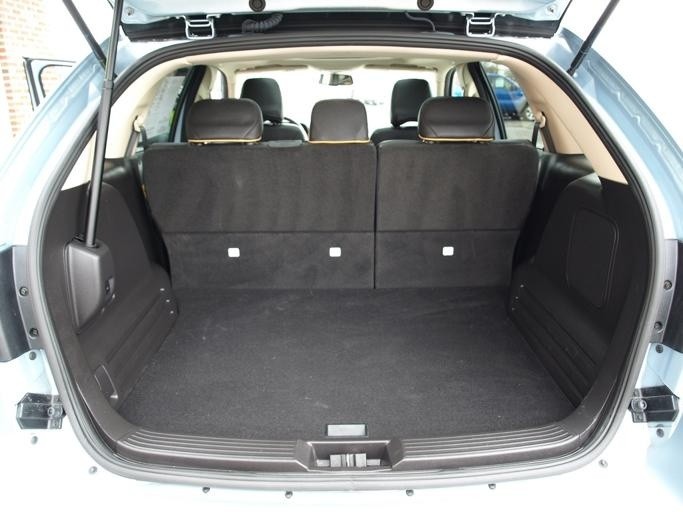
xmin=371 ymin=79 xmax=432 ymax=154
xmin=239 ymin=78 xmax=309 ymax=143
xmin=376 ymin=96 xmax=539 ymax=294
xmin=144 ymin=98 xmax=377 ymax=294
